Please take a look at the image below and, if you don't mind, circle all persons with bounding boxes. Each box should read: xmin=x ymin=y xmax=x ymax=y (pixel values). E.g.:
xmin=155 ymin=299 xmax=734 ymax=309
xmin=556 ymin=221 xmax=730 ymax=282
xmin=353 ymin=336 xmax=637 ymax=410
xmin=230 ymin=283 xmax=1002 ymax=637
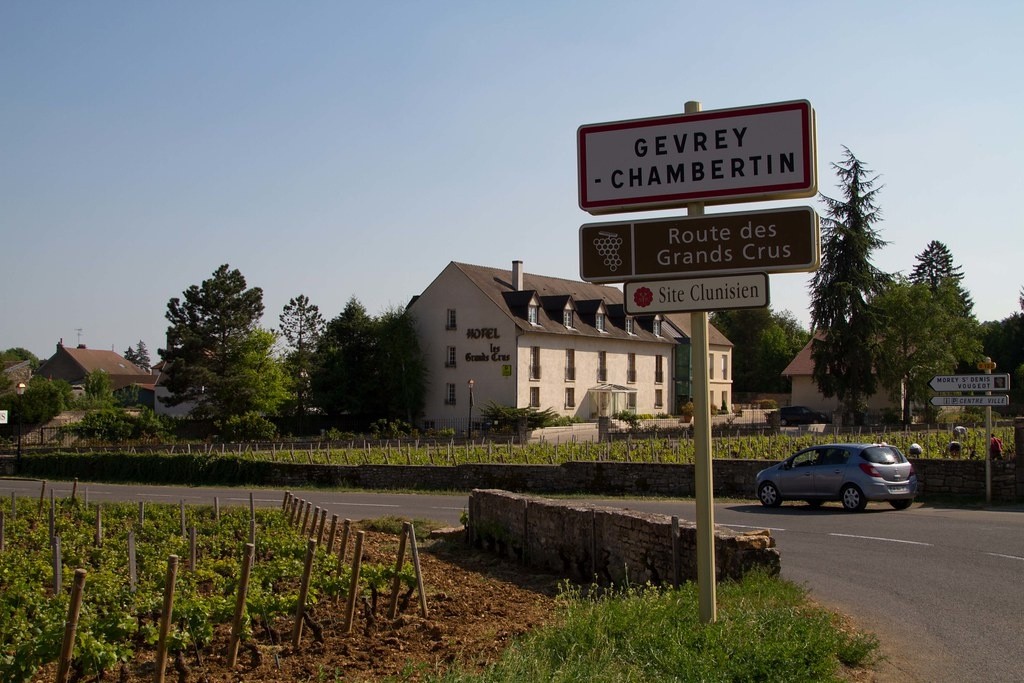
xmin=944 ymin=441 xmax=961 ymax=457
xmin=990 ymin=433 xmax=1003 ymax=460
xmin=968 ymin=451 xmax=979 ymax=460
xmin=909 ymin=443 xmax=923 ymax=458
xmin=953 ymin=426 xmax=967 ymax=440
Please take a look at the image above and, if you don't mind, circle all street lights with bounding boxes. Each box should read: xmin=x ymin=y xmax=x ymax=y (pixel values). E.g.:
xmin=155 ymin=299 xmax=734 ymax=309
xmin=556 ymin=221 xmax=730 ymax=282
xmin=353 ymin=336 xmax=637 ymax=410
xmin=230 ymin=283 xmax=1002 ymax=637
xmin=15 ymin=382 xmax=26 ymax=477
xmin=467 ymin=378 xmax=474 ymax=440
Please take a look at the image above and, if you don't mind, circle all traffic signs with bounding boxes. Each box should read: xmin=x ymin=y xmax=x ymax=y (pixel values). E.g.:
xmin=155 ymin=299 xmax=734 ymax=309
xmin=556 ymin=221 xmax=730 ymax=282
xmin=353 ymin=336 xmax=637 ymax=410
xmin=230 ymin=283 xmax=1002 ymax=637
xmin=930 ymin=395 xmax=1009 ymax=407
xmin=926 ymin=374 xmax=1011 ymax=393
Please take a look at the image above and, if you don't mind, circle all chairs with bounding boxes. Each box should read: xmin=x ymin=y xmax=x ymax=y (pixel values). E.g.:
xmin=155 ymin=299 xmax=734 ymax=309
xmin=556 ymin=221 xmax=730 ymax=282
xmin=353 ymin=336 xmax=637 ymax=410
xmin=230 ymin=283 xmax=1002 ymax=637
xmin=733 ymin=404 xmax=758 ymax=417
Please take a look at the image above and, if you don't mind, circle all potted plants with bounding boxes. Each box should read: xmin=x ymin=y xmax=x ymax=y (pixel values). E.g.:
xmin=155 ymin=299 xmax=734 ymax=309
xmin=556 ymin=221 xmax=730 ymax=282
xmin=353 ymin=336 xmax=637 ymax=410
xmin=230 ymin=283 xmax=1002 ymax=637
xmin=680 ymin=401 xmax=694 ymax=423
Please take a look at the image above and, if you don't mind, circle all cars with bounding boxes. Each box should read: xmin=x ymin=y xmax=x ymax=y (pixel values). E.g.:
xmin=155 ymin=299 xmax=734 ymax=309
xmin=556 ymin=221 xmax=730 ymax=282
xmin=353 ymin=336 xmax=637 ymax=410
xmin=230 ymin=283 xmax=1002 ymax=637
xmin=780 ymin=405 xmax=829 ymax=427
xmin=753 ymin=442 xmax=919 ymax=514
